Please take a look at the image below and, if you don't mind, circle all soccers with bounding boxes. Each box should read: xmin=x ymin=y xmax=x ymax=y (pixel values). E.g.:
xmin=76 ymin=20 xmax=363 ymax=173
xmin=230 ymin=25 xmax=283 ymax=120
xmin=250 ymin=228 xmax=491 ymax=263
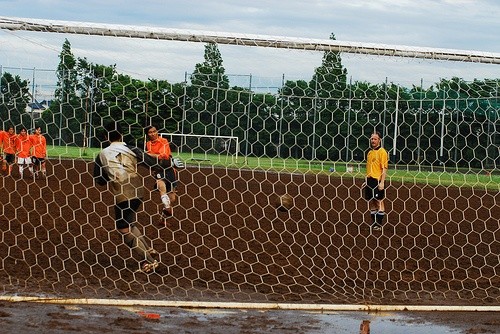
xmin=275 ymin=194 xmax=294 ymax=213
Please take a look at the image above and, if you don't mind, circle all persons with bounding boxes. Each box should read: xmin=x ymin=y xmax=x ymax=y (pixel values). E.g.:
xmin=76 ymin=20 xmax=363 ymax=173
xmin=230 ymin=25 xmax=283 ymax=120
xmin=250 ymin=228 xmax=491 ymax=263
xmin=93 ymin=122 xmax=185 ymax=272
xmin=143 ymin=125 xmax=178 ymax=227
xmin=0 ymin=125 xmax=48 ymax=180
xmin=365 ymin=132 xmax=390 ymax=230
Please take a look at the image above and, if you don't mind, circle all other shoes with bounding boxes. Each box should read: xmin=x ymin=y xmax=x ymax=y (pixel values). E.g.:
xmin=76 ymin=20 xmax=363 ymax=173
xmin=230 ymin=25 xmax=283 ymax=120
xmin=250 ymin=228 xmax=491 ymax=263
xmin=161 ymin=207 xmax=175 ymax=218
xmin=147 ymin=247 xmax=158 ymax=258
xmin=363 ymin=225 xmax=382 ymax=231
xmin=136 ymin=260 xmax=159 ymax=274
